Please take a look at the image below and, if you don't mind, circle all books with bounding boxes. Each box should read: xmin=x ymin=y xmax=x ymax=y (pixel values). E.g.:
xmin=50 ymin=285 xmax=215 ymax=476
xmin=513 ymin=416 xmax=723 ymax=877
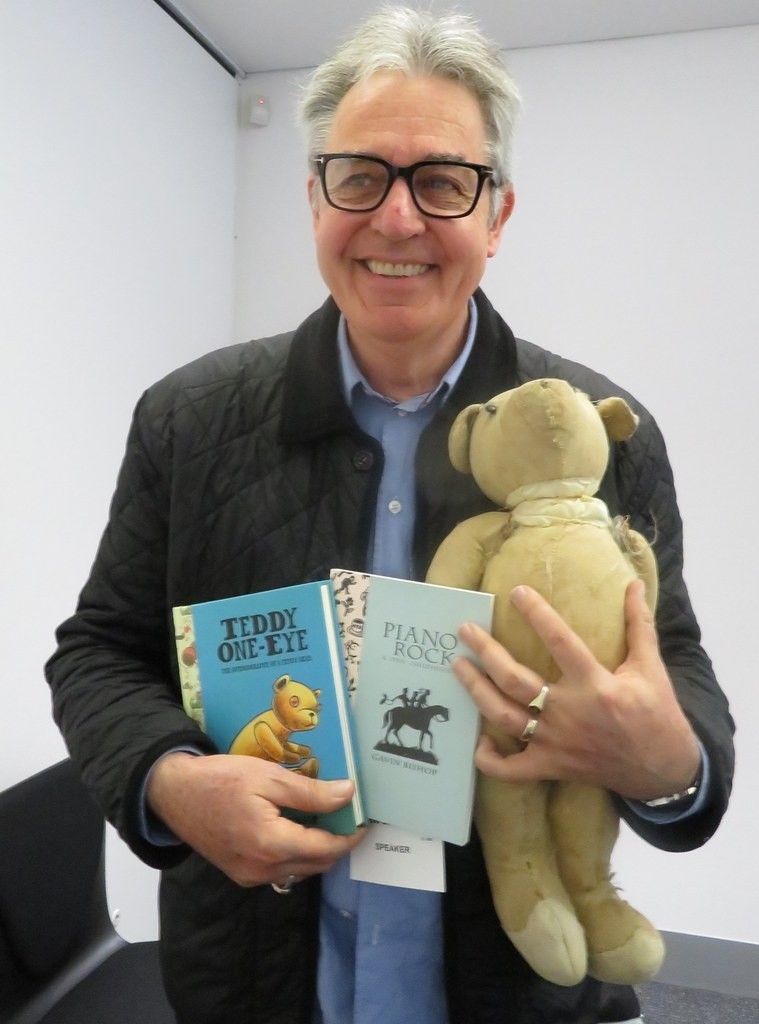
xmin=167 ymin=578 xmax=366 ymax=839
xmin=326 ymin=565 xmax=496 ymax=847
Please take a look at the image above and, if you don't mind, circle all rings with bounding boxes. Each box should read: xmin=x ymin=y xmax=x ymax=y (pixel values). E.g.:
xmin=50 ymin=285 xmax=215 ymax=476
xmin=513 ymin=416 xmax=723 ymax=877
xmin=271 ymin=876 xmax=293 ymax=894
xmin=526 ymin=685 xmax=549 ymax=714
xmin=517 ymin=716 xmax=538 ymax=742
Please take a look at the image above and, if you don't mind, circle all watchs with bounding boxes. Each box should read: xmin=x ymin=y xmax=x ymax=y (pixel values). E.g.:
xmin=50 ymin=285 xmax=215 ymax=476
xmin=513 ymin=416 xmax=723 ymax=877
xmin=639 ymin=776 xmax=701 ymax=809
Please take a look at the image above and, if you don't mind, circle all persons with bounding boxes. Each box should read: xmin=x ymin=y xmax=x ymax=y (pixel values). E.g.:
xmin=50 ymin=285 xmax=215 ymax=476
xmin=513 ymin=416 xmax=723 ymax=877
xmin=44 ymin=3 xmax=736 ymax=1024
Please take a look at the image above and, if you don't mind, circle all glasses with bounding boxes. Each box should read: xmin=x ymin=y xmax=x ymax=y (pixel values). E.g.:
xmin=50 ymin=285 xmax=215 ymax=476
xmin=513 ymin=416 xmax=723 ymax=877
xmin=313 ymin=153 xmax=500 ymax=219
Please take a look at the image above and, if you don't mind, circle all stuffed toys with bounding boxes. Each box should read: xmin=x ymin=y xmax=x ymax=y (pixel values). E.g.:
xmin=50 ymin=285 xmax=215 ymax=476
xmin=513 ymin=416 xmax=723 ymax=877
xmin=423 ymin=378 xmax=665 ymax=987
